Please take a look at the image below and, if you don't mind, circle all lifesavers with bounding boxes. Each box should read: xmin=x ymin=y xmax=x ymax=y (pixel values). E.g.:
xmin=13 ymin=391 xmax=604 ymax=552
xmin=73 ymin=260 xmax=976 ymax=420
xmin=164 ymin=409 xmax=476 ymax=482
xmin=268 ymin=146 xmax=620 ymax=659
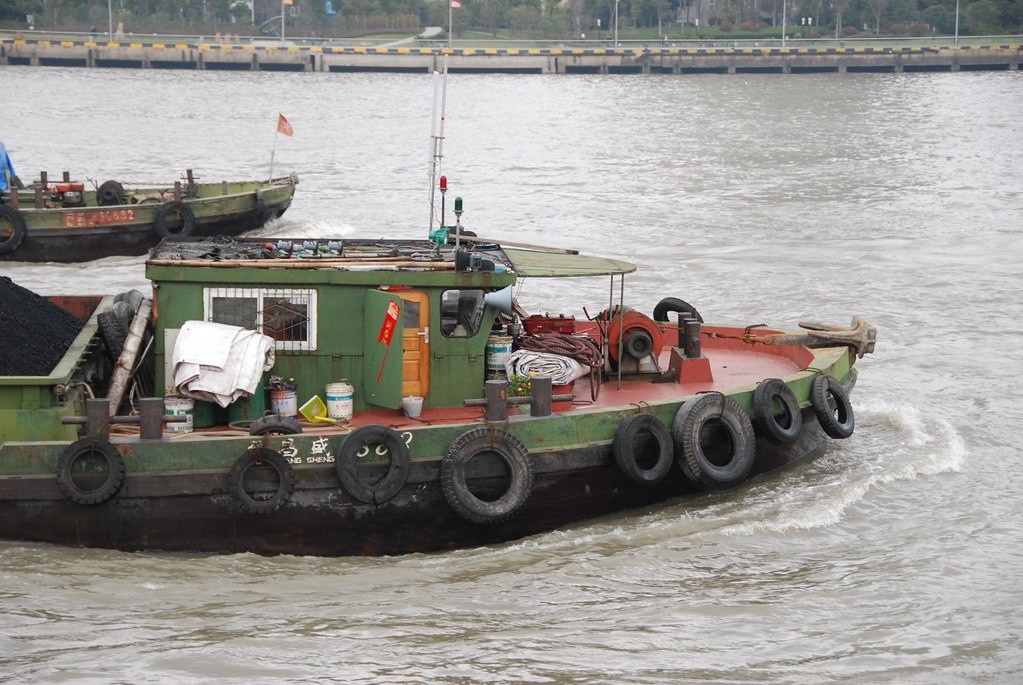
xmin=612 ymin=412 xmax=674 ymax=485
xmin=673 ymin=393 xmax=757 ymax=490
xmin=653 ymin=296 xmax=705 ymax=324
xmin=336 ymin=425 xmax=413 ymax=503
xmin=58 ymin=438 xmax=127 ymax=506
xmin=229 ymin=447 xmax=297 ymax=516
xmin=755 ymin=378 xmax=803 ymax=445
xmin=812 ymin=373 xmax=857 ymax=438
xmin=0 ymin=203 xmax=28 ymax=255
xmin=154 ymin=201 xmax=196 ymax=241
xmin=439 ymin=427 xmax=537 ymax=523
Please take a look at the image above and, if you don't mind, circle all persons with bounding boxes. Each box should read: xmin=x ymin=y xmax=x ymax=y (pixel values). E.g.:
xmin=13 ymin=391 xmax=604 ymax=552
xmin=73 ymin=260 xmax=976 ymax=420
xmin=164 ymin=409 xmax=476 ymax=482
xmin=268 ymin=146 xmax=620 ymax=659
xmin=90 ymin=25 xmax=98 ymax=41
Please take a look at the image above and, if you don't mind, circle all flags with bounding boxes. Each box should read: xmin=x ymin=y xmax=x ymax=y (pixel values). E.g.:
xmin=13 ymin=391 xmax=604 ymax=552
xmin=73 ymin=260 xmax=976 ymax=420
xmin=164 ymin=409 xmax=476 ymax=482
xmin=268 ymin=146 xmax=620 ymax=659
xmin=450 ymin=1 xmax=460 ymax=8
xmin=284 ymin=0 xmax=293 ymax=5
xmin=277 ymin=113 xmax=294 ymax=137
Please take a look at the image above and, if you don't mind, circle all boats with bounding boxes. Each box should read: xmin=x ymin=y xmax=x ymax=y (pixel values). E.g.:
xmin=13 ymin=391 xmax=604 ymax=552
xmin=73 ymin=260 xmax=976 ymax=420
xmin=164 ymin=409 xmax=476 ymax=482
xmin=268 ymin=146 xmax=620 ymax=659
xmin=0 ymin=53 xmax=877 ymax=558
xmin=0 ymin=140 xmax=299 ymax=264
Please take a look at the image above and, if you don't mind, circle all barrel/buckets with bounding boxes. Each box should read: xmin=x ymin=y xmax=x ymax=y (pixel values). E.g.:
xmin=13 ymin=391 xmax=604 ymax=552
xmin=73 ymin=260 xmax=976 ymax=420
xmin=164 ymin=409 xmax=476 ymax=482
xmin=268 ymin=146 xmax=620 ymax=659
xmin=485 ymin=335 xmax=513 ymax=381
xmin=326 ymin=384 xmax=353 ymax=422
xmin=166 ymin=376 xmax=265 ymax=433
xmin=402 ymin=397 xmax=424 ymax=418
xmin=270 ymin=387 xmax=297 ymax=420
